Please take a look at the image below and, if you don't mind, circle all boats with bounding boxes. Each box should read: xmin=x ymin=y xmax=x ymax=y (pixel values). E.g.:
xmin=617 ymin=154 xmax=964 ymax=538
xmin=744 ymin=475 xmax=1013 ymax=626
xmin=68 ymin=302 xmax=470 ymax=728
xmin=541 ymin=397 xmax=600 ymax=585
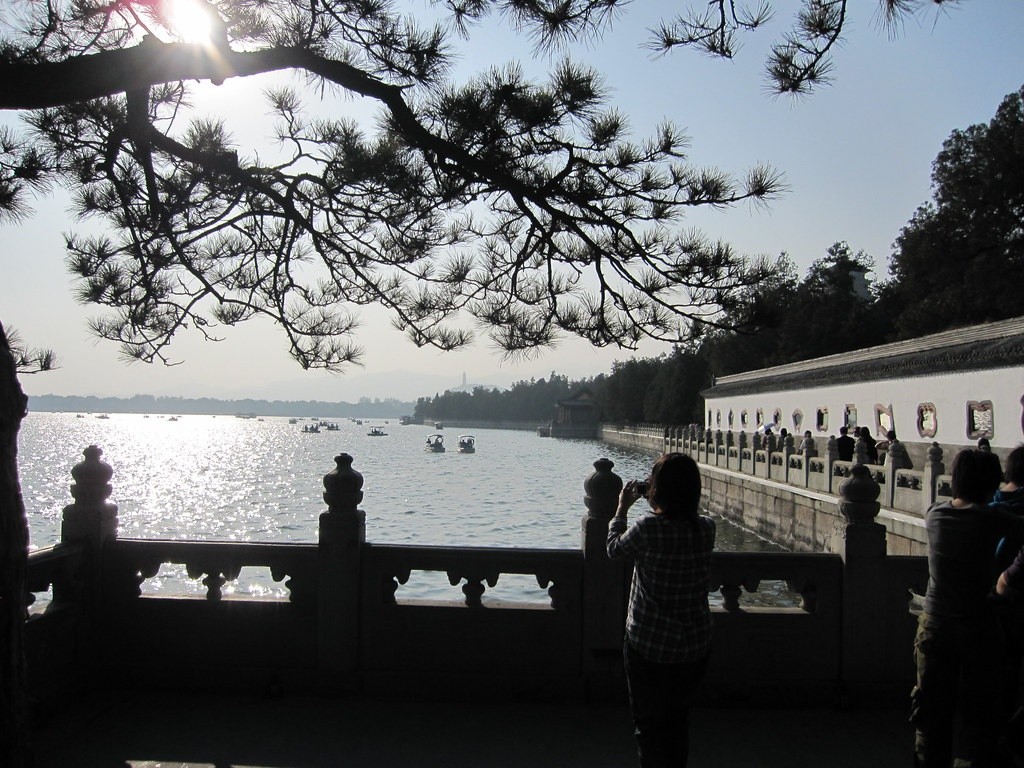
xmin=400 ymin=416 xmax=419 ymax=425
xmin=51 ymin=410 xmax=93 ymax=414
xmin=537 ymin=426 xmax=548 ymax=437
xmin=96 ymin=414 xmax=109 ymax=419
xmin=76 ymin=414 xmax=85 ymax=417
xmin=458 ymin=435 xmax=476 ymax=453
xmin=143 ymin=411 xmax=389 ymax=424
xmin=319 ymin=419 xmax=329 ymax=427
xmin=423 ymin=434 xmax=445 ymax=452
xmin=326 ymin=421 xmax=340 ymax=431
xmin=434 ymin=421 xmax=443 ymax=430
xmin=366 ymin=426 xmax=388 ymax=436
xmin=301 ymin=423 xmax=320 ymax=433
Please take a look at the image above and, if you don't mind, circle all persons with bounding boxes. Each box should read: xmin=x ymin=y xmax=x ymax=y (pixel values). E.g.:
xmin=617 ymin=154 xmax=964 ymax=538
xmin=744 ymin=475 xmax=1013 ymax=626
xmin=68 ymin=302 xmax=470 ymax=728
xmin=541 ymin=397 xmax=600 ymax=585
xmin=763 ymin=426 xmax=1024 ymax=768
xmin=606 ymin=451 xmax=717 ymax=768
xmin=304 ymin=419 xmax=474 ymax=448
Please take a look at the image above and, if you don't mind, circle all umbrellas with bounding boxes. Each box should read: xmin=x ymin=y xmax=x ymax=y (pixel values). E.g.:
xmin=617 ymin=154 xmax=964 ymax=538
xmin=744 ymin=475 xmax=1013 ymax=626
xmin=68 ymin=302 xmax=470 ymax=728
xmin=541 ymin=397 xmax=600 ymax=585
xmin=756 ymin=423 xmax=776 ymax=435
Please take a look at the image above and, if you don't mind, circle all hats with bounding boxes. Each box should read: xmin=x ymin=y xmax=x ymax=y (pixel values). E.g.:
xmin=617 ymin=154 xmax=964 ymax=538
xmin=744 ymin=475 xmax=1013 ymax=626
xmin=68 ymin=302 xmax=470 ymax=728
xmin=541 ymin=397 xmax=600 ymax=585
xmin=765 ymin=428 xmax=771 ymax=435
xmin=978 ymin=438 xmax=989 ymax=446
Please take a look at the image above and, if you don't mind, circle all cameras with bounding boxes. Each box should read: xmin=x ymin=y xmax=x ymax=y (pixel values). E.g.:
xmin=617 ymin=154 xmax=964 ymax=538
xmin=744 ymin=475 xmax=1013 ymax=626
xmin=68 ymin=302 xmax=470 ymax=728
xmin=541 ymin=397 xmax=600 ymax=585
xmin=634 ymin=482 xmax=650 ymax=495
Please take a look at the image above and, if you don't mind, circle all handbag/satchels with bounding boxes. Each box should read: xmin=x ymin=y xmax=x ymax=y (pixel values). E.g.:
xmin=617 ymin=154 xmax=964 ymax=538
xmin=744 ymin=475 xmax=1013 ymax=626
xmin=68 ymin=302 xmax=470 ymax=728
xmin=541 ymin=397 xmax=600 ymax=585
xmin=902 ymin=447 xmax=915 ymax=470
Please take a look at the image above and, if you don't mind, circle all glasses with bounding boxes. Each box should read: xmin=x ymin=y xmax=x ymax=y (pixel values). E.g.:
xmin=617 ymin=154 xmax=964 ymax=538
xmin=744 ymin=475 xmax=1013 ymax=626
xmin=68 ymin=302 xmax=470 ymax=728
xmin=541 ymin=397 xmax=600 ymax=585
xmin=853 ymin=431 xmax=858 ymax=433
